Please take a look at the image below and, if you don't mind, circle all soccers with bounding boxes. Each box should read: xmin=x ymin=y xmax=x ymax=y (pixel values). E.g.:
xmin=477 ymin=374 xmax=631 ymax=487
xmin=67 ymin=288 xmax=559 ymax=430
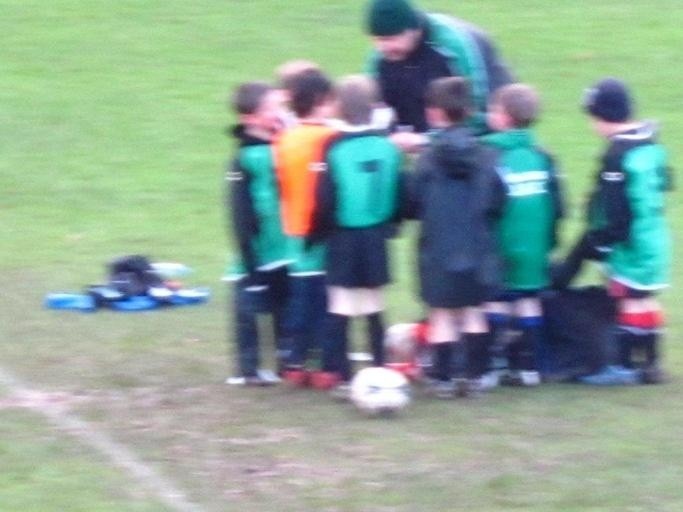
xmin=352 ymin=366 xmax=409 ymax=412
xmin=385 ymin=322 xmax=418 ymax=362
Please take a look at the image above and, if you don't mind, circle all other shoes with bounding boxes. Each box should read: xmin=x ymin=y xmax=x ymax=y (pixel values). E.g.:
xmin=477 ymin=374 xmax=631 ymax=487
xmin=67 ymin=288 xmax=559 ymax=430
xmin=225 ymin=346 xmax=660 ymax=390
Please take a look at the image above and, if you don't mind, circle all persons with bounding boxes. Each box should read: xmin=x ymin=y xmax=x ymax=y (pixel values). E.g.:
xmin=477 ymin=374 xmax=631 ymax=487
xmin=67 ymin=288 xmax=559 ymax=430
xmin=226 ymin=1 xmax=669 ymax=391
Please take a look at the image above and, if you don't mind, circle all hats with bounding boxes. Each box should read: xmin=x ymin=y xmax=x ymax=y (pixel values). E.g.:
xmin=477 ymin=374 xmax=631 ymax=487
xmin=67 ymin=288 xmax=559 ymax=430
xmin=366 ymin=1 xmax=419 ymax=36
xmin=582 ymin=80 xmax=630 ymax=123
